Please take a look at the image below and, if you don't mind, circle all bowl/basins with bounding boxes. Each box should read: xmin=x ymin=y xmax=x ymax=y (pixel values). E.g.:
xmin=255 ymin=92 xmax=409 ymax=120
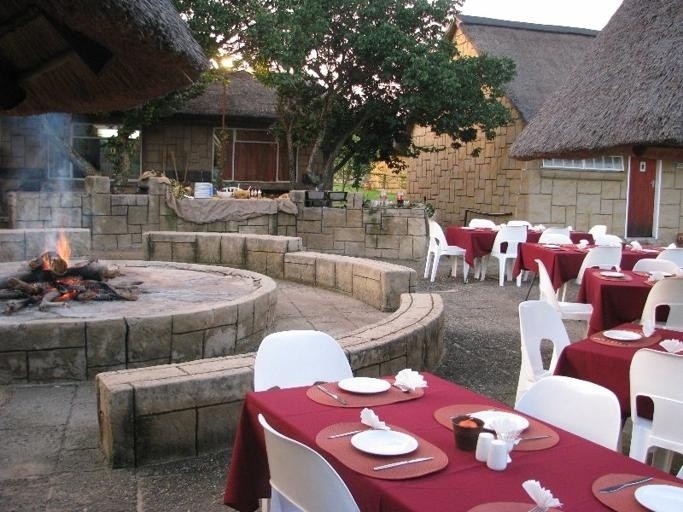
xmin=217 ymin=192 xmax=233 ymax=200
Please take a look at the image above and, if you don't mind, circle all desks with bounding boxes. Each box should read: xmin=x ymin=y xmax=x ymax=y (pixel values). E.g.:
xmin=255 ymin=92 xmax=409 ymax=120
xmin=445 ymin=224 xmax=594 ymax=278
xmin=513 ymin=242 xmax=680 ymax=293
xmin=553 ymin=322 xmax=682 ymax=425
xmin=576 ymin=267 xmax=658 ymax=339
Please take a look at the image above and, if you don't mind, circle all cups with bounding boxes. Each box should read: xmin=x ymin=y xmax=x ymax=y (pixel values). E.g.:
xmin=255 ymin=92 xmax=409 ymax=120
xmin=451 ymin=415 xmax=485 ymax=450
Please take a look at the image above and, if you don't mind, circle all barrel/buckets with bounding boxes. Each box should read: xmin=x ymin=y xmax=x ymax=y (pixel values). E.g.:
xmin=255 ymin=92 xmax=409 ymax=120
xmin=194 ymin=181 xmax=214 ymax=199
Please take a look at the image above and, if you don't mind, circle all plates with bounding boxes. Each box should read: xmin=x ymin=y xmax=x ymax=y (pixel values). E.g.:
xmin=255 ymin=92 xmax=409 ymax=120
xmin=542 ymin=244 xmax=560 ymax=248
xmin=460 ymin=227 xmax=476 ymax=230
xmin=649 ymin=270 xmax=673 ymax=277
xmin=599 ymin=271 xmax=625 ymax=278
xmin=633 ymin=484 xmax=682 ymax=511
xmin=602 ymin=329 xmax=642 ymax=341
xmin=468 ymin=409 xmax=530 ymax=437
xmin=351 ymin=429 xmax=419 ymax=457
xmin=338 ymin=376 xmax=391 ymax=396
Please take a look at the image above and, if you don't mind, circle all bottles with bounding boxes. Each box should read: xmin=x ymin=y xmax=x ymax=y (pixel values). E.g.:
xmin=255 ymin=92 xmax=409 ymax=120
xmin=475 ymin=432 xmax=494 ymax=462
xmin=396 ymin=192 xmax=404 ymax=206
xmin=251 ymin=189 xmax=262 ymax=199
xmin=486 ymin=439 xmax=507 ymax=470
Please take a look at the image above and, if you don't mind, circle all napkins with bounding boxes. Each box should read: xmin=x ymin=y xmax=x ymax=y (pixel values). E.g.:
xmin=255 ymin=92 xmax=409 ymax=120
xmin=659 ymin=339 xmax=682 ymax=355
xmin=489 ymin=419 xmax=523 ymax=452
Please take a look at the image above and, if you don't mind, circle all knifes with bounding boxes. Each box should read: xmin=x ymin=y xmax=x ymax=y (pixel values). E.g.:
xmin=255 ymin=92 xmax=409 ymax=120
xmin=317 ymin=384 xmax=348 ymax=405
xmin=597 ymin=475 xmax=655 ymax=494
xmin=373 ymin=456 xmax=434 ymax=470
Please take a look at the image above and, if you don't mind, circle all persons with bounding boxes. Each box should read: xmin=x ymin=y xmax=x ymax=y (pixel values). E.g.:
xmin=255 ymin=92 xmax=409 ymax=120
xmin=655 ymin=232 xmax=682 ymax=268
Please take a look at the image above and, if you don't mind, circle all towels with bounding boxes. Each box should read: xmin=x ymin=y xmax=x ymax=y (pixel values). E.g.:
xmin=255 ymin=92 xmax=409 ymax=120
xmin=391 ymin=368 xmax=429 ymax=392
xmin=360 ymin=407 xmax=391 ymax=431
xmin=522 ymin=479 xmax=564 ymax=509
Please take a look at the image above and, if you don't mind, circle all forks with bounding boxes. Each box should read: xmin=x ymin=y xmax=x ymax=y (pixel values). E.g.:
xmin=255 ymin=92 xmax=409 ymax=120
xmin=527 ymin=504 xmax=549 ymax=512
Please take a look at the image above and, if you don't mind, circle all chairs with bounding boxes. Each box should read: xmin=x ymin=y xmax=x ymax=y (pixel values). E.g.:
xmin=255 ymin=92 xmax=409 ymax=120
xmin=587 ymin=224 xmax=607 ymax=244
xmin=514 ymin=299 xmax=573 ymax=409
xmin=480 ymin=225 xmax=528 ymax=287
xmin=469 ymin=219 xmax=515 ymax=281
xmin=595 ymin=233 xmax=623 ymax=249
xmin=632 ymin=258 xmax=679 ymax=274
xmin=639 ymin=277 xmax=680 ymax=333
xmin=542 ymin=234 xmax=574 ymax=302
xmin=656 ymin=248 xmax=681 ymax=268
xmin=561 ymin=243 xmax=623 ymax=302
xmin=533 ymin=258 xmax=594 ymax=339
xmin=254 ymin=330 xmax=354 ymax=512
xmin=258 ymin=414 xmax=361 ymax=512
xmin=538 ymin=227 xmax=570 ymax=245
xmin=507 ymin=220 xmax=535 ymax=281
xmin=629 ymin=348 xmax=683 ymax=480
xmin=426 ymin=218 xmax=468 ymax=284
xmin=513 ymin=375 xmax=623 ymax=456
